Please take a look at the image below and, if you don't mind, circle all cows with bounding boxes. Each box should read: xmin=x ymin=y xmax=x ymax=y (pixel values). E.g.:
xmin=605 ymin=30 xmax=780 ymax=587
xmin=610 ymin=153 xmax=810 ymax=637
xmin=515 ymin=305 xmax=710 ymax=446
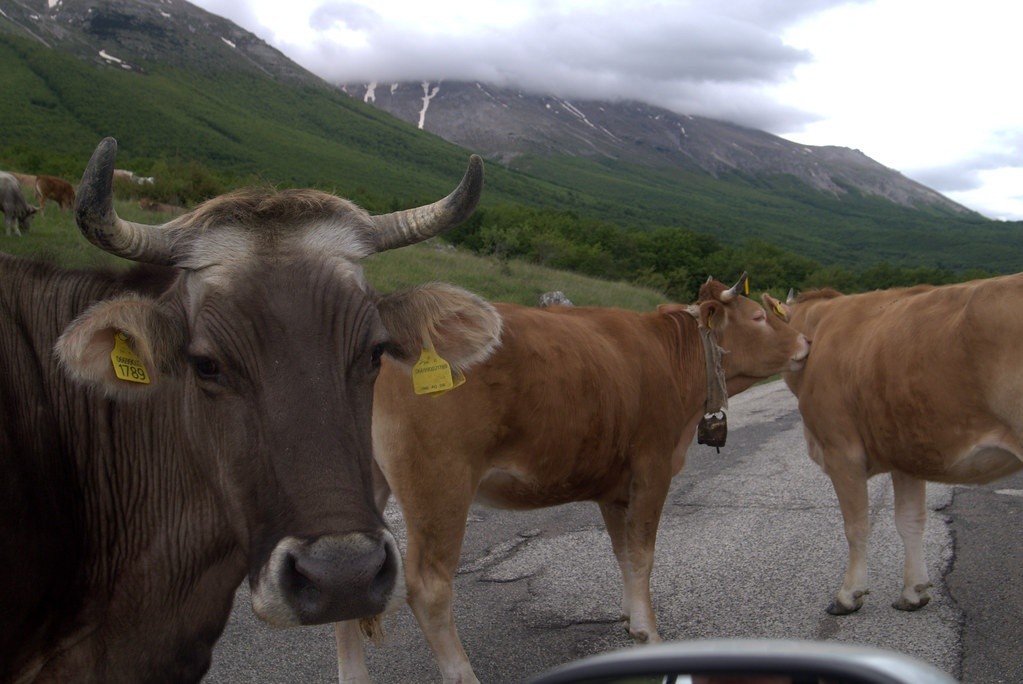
xmin=3 ymin=169 xmax=155 ymax=233
xmin=2 ymin=136 xmax=486 ymax=684
xmin=758 ymin=274 xmax=1022 ymax=617
xmin=334 ymin=272 xmax=814 ymax=684
